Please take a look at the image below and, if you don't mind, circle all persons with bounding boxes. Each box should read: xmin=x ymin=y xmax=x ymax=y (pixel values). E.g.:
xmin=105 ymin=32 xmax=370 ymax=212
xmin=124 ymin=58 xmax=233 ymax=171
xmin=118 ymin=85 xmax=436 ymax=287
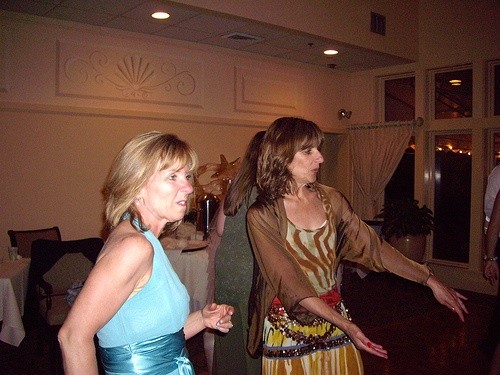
xmin=483 ymin=165 xmax=500 ymax=287
xmin=57 ymin=130 xmax=235 ymax=375
xmin=244 ymin=116 xmax=468 ymax=375
xmin=211 ymin=130 xmax=267 ymax=375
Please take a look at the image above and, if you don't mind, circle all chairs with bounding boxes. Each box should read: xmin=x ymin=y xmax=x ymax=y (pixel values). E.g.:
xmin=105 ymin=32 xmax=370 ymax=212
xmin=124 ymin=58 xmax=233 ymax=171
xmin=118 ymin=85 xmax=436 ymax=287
xmin=28 ymin=238 xmax=104 ymax=362
xmin=8 ymin=226 xmax=61 ymax=310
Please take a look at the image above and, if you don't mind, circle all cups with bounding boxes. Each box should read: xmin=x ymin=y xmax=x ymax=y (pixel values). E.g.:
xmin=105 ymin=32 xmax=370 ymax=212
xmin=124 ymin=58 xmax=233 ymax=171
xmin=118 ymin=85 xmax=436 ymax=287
xmin=8 ymin=247 xmax=18 ymax=261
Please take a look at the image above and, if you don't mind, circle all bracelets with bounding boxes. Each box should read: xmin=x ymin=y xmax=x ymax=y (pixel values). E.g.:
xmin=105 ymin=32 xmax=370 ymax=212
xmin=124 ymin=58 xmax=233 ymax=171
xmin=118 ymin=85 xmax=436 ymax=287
xmin=484 ymin=255 xmax=498 ymax=261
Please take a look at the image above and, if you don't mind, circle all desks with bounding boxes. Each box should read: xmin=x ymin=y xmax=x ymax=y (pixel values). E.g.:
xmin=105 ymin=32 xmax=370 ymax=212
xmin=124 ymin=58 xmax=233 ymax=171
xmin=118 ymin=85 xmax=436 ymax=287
xmin=0 ymin=258 xmax=31 ymax=347
xmin=160 ymin=237 xmax=209 ymax=310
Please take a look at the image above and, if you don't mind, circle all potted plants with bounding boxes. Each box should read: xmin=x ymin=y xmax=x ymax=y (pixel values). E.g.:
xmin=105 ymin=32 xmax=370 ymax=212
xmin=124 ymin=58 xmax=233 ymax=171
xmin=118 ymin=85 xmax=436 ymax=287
xmin=373 ymin=197 xmax=435 ymax=264
